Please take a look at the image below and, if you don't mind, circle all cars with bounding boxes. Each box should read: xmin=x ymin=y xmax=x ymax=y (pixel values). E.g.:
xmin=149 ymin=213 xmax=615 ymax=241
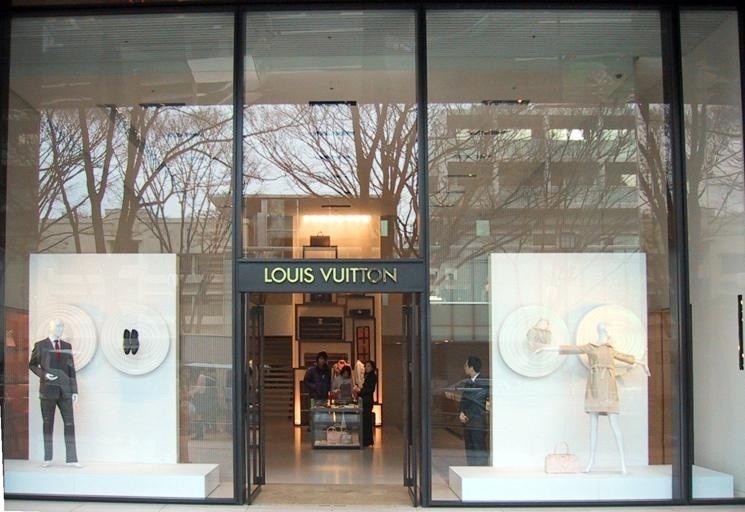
xmin=180 ymin=362 xmax=234 ymax=435
xmin=431 ymin=377 xmax=523 ymax=417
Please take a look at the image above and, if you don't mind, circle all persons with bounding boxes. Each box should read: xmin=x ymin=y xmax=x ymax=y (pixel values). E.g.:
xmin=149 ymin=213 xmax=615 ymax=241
xmin=354 ymin=360 xmax=366 ymax=390
xmin=178 ymin=366 xmax=230 ymax=440
xmin=457 ymin=355 xmax=488 ymax=464
xmin=28 ymin=318 xmax=84 ymax=468
xmin=333 ymin=365 xmax=353 ymax=433
xmin=357 ymin=360 xmax=377 ymax=446
xmin=533 ymin=321 xmax=650 ymax=474
xmin=332 ymin=359 xmax=353 ymax=378
xmin=304 ymin=351 xmax=332 ymax=432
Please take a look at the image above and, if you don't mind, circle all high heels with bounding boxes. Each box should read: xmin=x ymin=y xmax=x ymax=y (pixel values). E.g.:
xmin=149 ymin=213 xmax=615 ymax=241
xmin=364 ymin=441 xmax=374 ymax=447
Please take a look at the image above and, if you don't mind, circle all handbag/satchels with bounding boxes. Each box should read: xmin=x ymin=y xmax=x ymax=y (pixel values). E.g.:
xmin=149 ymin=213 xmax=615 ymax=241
xmin=529 ymin=319 xmax=551 ymax=346
xmin=326 ymin=423 xmax=353 ymax=444
xmin=545 ymin=441 xmax=578 ymax=474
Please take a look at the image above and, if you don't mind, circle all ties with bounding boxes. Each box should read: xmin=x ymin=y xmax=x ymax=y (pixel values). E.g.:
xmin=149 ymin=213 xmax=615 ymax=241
xmin=54 ymin=340 xmax=61 ymax=361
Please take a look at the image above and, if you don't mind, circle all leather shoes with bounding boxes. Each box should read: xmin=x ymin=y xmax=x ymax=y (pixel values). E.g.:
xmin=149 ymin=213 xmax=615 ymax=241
xmin=123 ymin=329 xmax=139 ymax=355
